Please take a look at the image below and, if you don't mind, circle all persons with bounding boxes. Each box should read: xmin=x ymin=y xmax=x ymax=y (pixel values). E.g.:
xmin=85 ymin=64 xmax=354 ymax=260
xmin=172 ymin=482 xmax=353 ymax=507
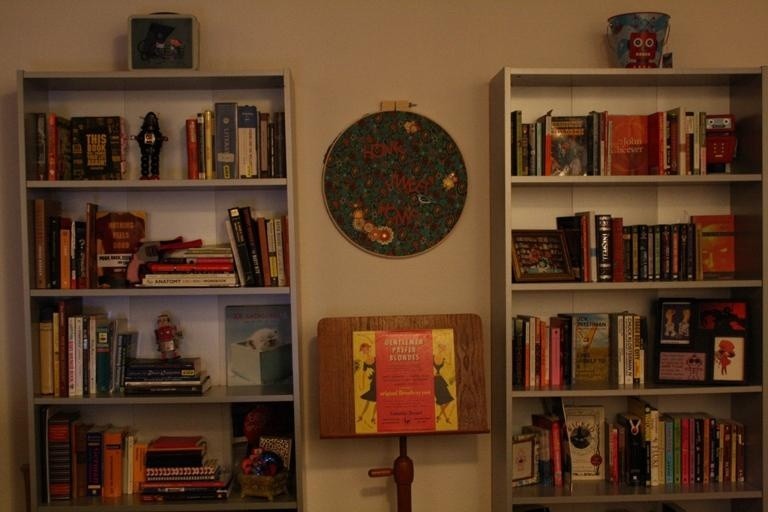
xmin=154 ymin=315 xmax=182 ymax=358
xmin=432 ymin=340 xmax=454 ymax=426
xmin=132 ymin=112 xmax=168 ymax=180
xmin=706 ymin=115 xmax=737 ymax=172
xmin=356 ymin=340 xmax=377 ymax=427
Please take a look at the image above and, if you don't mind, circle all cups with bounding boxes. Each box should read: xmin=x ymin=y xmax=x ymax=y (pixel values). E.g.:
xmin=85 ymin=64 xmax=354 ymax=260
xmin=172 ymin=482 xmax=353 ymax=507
xmin=607 ymin=12 xmax=670 ymax=68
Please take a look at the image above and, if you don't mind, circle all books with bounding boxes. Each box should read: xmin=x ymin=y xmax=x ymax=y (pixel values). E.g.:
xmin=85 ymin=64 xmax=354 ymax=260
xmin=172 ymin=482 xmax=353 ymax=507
xmin=513 ymin=110 xmax=588 ymax=176
xmin=26 ymin=112 xmax=126 ymax=181
xmin=224 ymin=305 xmax=291 ymax=396
xmin=138 ymin=437 xmax=235 ymax=499
xmin=186 ymin=104 xmax=287 ymax=179
xmin=514 ymin=397 xmax=646 ymax=486
xmin=627 ymin=396 xmax=744 ymax=486
xmin=513 ymin=311 xmax=648 ymax=390
xmin=135 ymin=206 xmax=288 ymax=288
xmin=231 ymin=401 xmax=290 ymax=500
xmin=35 ymin=405 xmax=146 ymax=499
xmin=588 ymin=108 xmax=707 ymax=176
xmin=514 ymin=210 xmax=625 ymax=283
xmin=39 ymin=297 xmax=137 ymax=398
xmin=624 ymin=215 xmax=759 ymax=281
xmin=651 ymin=297 xmax=751 ymax=385
xmin=33 ymin=198 xmax=148 ymax=289
xmin=123 ymin=356 xmax=211 ymax=396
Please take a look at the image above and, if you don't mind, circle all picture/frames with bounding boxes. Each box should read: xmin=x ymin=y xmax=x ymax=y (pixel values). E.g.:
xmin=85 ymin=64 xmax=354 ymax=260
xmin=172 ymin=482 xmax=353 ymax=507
xmin=710 ymin=333 xmax=750 ymax=386
xmin=655 ymin=297 xmax=697 ymax=352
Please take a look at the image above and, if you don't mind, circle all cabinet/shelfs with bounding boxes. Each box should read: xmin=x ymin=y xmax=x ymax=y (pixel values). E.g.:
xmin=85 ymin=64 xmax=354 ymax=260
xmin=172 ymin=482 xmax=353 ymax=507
xmin=15 ymin=69 xmax=307 ymax=512
xmin=490 ymin=66 xmax=768 ymax=512
